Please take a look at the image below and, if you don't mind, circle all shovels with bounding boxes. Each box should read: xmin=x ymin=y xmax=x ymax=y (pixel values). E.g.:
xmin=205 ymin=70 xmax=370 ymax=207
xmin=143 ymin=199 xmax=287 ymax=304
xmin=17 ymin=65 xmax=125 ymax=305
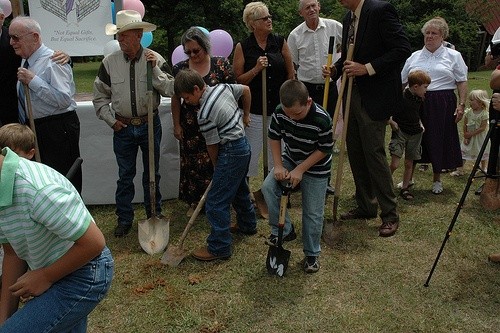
xmin=138 ymin=59 xmax=171 ymax=255
xmin=161 ymin=180 xmax=213 ymax=265
xmin=265 ymin=179 xmax=295 ymax=277
xmin=254 ymin=67 xmax=270 ymax=220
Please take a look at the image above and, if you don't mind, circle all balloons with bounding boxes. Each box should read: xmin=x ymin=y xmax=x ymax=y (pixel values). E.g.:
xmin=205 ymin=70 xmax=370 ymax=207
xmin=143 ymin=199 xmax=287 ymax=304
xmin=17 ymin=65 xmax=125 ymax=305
xmin=103 ymin=0 xmax=153 ymax=61
xmin=171 ymin=26 xmax=233 ymax=67
xmin=0 ymin=0 xmax=12 ymax=17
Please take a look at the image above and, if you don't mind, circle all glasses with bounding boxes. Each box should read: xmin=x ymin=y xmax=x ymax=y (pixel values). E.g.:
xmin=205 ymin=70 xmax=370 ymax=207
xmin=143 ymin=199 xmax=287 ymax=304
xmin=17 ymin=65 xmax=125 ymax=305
xmin=9 ymin=31 xmax=33 ymax=42
xmin=253 ymin=15 xmax=273 ymax=22
xmin=184 ymin=46 xmax=204 ymax=55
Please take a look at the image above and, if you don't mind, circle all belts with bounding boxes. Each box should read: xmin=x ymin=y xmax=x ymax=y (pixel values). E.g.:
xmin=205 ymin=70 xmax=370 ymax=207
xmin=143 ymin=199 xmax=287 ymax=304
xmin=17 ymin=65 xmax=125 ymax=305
xmin=302 ymin=81 xmax=335 ymax=89
xmin=115 ymin=110 xmax=159 ymax=125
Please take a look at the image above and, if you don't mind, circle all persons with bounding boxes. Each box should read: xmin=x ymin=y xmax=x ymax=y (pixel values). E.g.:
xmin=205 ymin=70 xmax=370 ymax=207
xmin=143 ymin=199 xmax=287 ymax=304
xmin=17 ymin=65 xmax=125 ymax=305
xmin=174 ymin=66 xmax=258 ymax=262
xmin=484 ymin=24 xmax=500 ymax=263
xmin=385 ymin=72 xmax=431 ymax=200
xmin=0 ymin=8 xmax=74 ymax=127
xmin=284 ymin=0 xmax=348 ymax=195
xmin=7 ymin=15 xmax=82 ymax=211
xmin=170 ymin=23 xmax=241 ymax=218
xmin=0 ymin=122 xmax=39 ymax=303
xmin=95 ymin=10 xmax=178 ymax=236
xmin=260 ymin=78 xmax=334 ymax=273
xmin=450 ymin=89 xmax=492 ymax=178
xmin=318 ymin=0 xmax=404 ymax=236
xmin=1 ymin=144 xmax=113 ymax=332
xmin=234 ymin=1 xmax=296 ymax=214
xmin=398 ymin=15 xmax=469 ymax=194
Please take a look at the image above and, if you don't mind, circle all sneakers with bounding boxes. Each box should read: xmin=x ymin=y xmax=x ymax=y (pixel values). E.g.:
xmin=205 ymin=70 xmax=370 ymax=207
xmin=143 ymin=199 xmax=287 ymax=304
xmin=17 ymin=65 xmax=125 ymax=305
xmin=303 ymin=255 xmax=320 ymax=273
xmin=264 ymin=224 xmax=296 ymax=246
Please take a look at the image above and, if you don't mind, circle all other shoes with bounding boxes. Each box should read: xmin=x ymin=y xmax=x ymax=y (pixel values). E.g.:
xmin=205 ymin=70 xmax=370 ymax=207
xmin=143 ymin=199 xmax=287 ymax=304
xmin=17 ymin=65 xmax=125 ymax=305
xmin=114 ymin=224 xmax=132 ymax=237
xmin=396 ymin=179 xmax=414 ymax=188
xmin=229 ymin=223 xmax=243 ymax=233
xmin=450 ymin=170 xmax=464 ymax=176
xmin=474 ymin=168 xmax=488 ymax=178
xmin=432 ymin=181 xmax=444 ymax=194
xmin=186 ymin=207 xmax=194 ymax=218
xmin=151 ymin=213 xmax=174 ymax=225
xmin=192 ymin=247 xmax=219 ymax=260
xmin=475 ymin=184 xmax=484 ymax=195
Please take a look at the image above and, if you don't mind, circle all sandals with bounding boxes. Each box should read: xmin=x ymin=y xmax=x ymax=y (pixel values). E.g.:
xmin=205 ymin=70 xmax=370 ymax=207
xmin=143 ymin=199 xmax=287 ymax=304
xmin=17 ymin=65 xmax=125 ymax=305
xmin=400 ymin=187 xmax=415 ymax=201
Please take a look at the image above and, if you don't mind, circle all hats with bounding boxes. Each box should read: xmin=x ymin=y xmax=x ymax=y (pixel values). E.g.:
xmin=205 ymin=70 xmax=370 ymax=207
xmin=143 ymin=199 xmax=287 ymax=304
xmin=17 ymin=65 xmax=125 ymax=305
xmin=105 ymin=10 xmax=156 ymax=36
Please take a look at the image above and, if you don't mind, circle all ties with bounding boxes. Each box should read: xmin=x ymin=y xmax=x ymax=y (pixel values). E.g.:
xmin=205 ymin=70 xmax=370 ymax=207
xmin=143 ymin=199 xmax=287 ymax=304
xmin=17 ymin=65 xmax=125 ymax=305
xmin=18 ymin=60 xmax=29 ymax=125
xmin=347 ymin=12 xmax=356 ymax=48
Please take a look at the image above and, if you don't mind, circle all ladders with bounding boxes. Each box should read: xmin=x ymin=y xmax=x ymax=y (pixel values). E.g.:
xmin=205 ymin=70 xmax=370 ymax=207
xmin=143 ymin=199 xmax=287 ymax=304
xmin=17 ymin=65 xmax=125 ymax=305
xmin=322 ymin=76 xmax=353 ymax=244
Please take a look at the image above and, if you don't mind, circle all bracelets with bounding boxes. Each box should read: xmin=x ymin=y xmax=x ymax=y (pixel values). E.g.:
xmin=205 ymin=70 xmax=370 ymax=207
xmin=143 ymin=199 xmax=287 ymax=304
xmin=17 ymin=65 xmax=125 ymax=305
xmin=459 ymin=102 xmax=466 ymax=108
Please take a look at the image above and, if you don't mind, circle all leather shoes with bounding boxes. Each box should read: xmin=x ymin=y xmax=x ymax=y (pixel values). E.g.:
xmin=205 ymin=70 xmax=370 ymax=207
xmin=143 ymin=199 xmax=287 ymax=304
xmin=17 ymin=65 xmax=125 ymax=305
xmin=341 ymin=209 xmax=369 ymax=220
xmin=380 ymin=219 xmax=399 ymax=237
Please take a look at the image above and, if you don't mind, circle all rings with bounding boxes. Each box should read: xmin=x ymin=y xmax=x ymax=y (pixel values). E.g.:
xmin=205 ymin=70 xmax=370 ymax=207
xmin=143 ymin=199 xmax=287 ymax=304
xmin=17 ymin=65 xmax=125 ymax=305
xmin=348 ymin=69 xmax=350 ymax=72
